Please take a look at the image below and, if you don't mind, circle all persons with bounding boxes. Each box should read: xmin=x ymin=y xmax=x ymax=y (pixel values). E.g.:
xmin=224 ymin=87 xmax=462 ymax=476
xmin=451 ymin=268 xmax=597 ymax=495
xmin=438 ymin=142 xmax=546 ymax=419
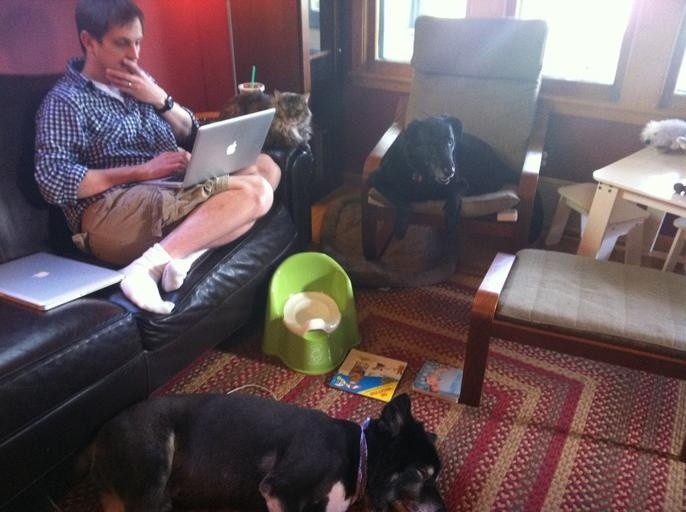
xmin=32 ymin=0 xmax=282 ymax=315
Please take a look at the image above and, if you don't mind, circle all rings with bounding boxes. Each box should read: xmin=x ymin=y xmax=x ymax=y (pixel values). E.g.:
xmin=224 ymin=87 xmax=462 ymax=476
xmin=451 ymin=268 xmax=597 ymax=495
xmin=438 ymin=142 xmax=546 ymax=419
xmin=127 ymin=81 xmax=131 ymax=88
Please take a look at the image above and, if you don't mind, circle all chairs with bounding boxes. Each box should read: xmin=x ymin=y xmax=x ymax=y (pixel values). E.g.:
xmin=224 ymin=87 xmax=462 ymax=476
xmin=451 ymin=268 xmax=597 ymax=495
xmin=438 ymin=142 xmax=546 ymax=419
xmin=355 ymin=13 xmax=556 ymax=266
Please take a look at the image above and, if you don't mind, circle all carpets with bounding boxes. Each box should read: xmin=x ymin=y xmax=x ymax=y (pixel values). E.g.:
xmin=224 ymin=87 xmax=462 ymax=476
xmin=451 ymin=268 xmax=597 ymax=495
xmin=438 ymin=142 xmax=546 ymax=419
xmin=318 ymin=193 xmax=458 ymax=290
xmin=29 ymin=256 xmax=685 ymax=512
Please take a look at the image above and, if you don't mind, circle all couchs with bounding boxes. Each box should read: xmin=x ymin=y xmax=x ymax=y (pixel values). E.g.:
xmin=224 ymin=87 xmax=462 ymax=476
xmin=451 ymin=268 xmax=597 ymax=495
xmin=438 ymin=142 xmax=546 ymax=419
xmin=0 ymin=62 xmax=323 ymax=512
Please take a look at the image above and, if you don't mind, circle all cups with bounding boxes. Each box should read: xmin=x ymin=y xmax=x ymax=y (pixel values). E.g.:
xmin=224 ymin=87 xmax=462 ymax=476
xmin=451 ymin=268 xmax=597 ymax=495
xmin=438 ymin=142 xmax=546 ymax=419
xmin=237 ymin=82 xmax=266 ymax=94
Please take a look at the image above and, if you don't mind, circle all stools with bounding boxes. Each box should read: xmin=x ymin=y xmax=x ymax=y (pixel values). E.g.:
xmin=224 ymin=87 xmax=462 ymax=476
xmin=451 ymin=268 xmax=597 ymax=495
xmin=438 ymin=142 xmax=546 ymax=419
xmin=544 ymin=180 xmax=652 ymax=267
xmin=455 ymin=246 xmax=685 ymax=465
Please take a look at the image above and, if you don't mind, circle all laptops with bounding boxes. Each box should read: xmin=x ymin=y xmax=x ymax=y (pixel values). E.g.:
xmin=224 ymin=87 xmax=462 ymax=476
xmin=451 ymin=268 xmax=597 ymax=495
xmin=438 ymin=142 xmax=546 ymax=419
xmin=137 ymin=108 xmax=276 ymax=189
xmin=0 ymin=251 xmax=125 ymax=312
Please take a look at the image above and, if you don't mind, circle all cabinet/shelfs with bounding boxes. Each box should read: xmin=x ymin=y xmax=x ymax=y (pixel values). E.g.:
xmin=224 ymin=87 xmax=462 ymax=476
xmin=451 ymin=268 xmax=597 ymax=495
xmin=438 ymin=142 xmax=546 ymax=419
xmin=228 ymin=0 xmax=344 ymax=194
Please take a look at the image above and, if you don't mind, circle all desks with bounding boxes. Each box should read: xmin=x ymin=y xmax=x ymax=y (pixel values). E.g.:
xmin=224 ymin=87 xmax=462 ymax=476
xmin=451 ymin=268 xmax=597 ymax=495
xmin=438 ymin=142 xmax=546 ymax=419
xmin=572 ymin=140 xmax=685 ymax=273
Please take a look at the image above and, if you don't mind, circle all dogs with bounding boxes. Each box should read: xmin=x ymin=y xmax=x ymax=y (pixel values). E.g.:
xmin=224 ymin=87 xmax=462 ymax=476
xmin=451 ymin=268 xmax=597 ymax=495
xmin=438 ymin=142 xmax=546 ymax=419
xmin=366 ymin=114 xmax=545 ymax=245
xmin=85 ymin=391 xmax=448 ymax=512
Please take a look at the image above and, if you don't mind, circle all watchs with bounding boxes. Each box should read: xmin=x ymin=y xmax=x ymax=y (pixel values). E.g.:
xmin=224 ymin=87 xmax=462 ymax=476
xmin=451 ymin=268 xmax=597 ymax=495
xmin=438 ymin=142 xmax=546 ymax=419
xmin=156 ymin=93 xmax=174 ymax=116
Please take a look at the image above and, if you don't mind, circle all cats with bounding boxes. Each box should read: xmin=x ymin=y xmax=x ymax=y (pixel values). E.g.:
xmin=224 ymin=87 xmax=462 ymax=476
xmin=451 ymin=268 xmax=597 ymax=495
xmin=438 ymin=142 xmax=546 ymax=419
xmin=217 ymin=88 xmax=312 ymax=149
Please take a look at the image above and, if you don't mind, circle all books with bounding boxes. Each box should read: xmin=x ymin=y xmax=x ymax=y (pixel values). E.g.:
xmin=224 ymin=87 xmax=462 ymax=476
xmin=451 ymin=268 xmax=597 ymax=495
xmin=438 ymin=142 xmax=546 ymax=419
xmin=327 ymin=346 xmax=407 ymax=404
xmin=413 ymin=359 xmax=464 ymax=405
